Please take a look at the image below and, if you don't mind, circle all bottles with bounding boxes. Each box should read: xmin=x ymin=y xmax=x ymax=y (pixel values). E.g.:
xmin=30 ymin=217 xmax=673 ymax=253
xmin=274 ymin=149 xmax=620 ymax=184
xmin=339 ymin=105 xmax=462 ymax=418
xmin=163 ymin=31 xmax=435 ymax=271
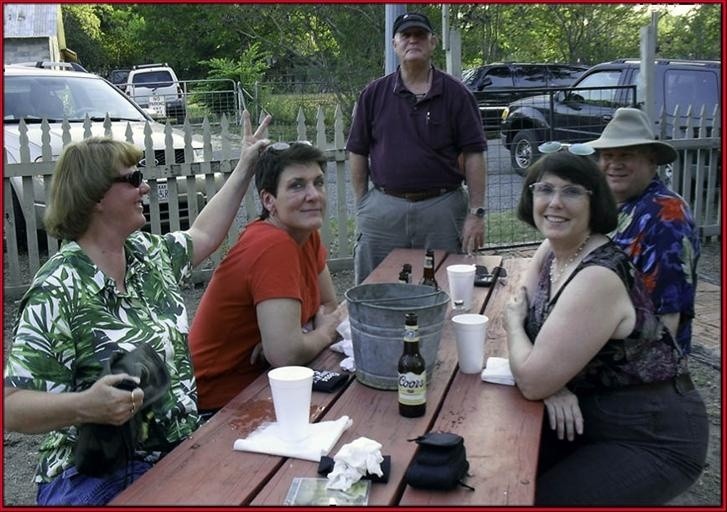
xmin=419 ymin=249 xmax=437 ymax=291
xmin=396 ymin=312 xmax=427 ymax=418
xmin=397 ymin=263 xmax=412 ymax=283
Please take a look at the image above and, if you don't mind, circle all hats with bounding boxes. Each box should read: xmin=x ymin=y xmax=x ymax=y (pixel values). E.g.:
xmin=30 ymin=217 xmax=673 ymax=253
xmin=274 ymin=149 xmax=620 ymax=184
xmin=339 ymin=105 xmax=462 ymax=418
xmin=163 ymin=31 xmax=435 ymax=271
xmin=583 ymin=107 xmax=677 ymax=165
xmin=392 ymin=12 xmax=435 ymax=42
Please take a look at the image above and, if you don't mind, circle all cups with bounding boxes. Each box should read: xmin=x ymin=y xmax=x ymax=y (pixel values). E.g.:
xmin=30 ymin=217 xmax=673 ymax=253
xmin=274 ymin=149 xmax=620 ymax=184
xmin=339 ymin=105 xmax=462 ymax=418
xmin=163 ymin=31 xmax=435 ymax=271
xmin=267 ymin=365 xmax=313 ymax=436
xmin=446 ymin=263 xmax=476 ymax=311
xmin=451 ymin=312 xmax=489 ymax=375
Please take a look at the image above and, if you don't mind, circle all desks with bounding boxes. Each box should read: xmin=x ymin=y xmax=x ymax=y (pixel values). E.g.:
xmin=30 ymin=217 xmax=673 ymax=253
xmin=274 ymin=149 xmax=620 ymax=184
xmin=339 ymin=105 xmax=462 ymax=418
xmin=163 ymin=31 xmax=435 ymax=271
xmin=99 ymin=244 xmax=550 ymax=507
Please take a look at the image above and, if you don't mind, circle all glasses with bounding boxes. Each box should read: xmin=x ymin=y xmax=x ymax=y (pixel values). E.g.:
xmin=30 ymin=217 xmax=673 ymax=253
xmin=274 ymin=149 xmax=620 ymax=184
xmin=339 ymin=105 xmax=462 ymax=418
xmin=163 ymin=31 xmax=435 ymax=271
xmin=474 ymin=265 xmax=507 ymax=286
xmin=108 ymin=168 xmax=145 ymax=187
xmin=528 ymin=182 xmax=594 ymax=200
xmin=257 ymin=140 xmax=312 ymax=166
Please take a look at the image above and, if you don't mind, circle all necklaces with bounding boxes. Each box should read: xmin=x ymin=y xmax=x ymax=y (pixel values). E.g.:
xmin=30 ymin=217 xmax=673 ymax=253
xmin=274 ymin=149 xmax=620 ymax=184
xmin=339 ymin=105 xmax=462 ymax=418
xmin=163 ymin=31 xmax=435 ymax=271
xmin=547 ymin=235 xmax=589 ymax=284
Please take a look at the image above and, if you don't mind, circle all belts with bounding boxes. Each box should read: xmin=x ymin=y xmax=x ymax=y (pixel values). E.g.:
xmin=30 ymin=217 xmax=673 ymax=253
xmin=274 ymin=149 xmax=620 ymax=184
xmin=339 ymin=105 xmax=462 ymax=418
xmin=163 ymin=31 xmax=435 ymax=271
xmin=373 ymin=183 xmax=456 ymax=202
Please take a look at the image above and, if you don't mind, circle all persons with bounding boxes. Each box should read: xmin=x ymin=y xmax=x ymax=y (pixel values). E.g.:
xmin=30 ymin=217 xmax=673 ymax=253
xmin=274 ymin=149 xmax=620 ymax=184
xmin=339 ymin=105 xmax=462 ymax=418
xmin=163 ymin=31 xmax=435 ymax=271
xmin=188 ymin=144 xmax=348 ymax=423
xmin=503 ymin=148 xmax=708 ymax=509
xmin=581 ymin=107 xmax=701 ymax=363
xmin=346 ymin=13 xmax=487 ymax=287
xmin=3 ymin=109 xmax=273 ymax=508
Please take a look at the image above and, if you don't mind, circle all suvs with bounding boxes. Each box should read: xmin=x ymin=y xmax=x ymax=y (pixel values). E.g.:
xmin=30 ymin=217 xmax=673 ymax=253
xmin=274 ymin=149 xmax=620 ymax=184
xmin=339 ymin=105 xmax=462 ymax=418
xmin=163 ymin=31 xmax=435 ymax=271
xmin=2 ymin=57 xmax=232 ymax=250
xmin=106 ymin=61 xmax=189 ymax=124
xmin=455 ymin=61 xmax=602 ymax=139
xmin=498 ymin=56 xmax=721 ymax=180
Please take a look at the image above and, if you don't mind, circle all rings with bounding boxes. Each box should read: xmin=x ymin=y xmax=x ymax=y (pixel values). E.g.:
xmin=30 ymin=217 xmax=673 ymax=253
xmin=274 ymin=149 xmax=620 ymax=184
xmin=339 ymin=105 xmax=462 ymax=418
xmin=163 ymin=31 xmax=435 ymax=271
xmin=130 ymin=393 xmax=136 ymax=418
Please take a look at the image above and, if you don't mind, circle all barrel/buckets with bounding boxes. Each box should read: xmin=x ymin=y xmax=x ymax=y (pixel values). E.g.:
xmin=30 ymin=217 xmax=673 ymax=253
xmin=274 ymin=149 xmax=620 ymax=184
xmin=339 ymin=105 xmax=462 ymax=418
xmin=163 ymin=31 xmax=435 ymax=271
xmin=344 ymin=283 xmax=452 ymax=392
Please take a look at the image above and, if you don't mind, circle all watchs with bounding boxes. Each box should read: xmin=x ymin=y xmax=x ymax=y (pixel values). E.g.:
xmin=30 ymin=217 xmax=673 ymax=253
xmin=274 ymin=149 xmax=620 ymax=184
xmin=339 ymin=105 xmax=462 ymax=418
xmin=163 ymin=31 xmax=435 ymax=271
xmin=470 ymin=207 xmax=485 ymax=218
